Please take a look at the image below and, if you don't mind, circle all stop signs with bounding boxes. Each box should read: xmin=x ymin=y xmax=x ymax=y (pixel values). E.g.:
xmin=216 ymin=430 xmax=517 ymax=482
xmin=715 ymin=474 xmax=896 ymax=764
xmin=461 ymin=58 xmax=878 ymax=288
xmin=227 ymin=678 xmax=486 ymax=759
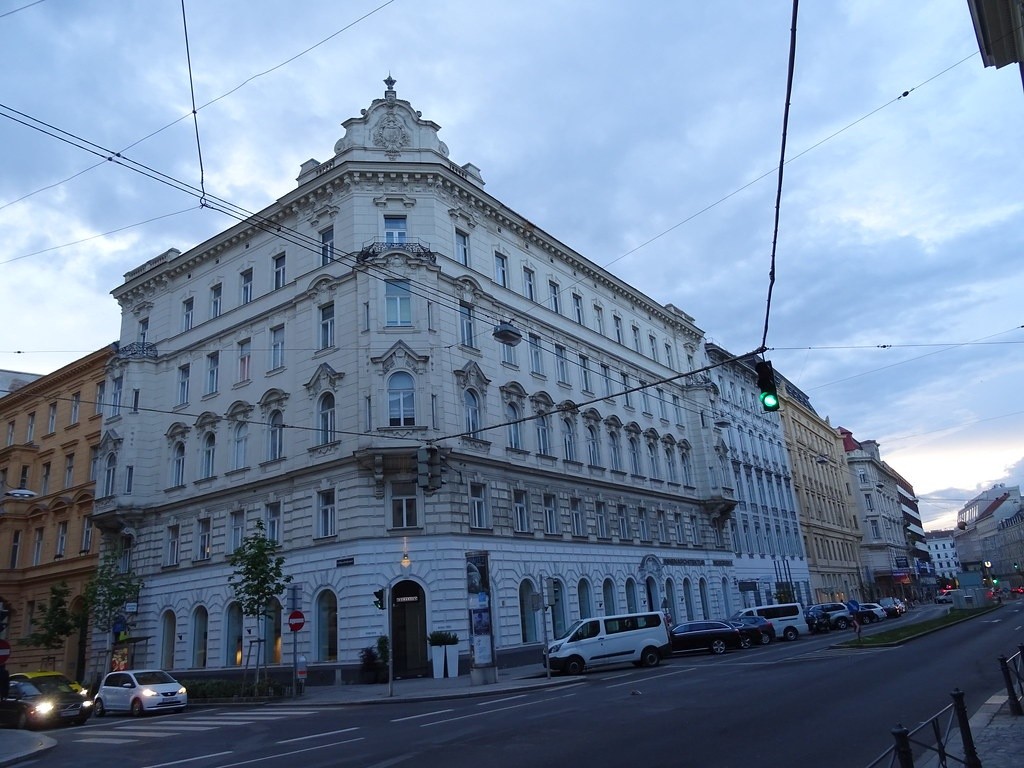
xmin=0 ymin=640 xmax=10 ymax=666
xmin=288 ymin=611 xmax=304 ymax=631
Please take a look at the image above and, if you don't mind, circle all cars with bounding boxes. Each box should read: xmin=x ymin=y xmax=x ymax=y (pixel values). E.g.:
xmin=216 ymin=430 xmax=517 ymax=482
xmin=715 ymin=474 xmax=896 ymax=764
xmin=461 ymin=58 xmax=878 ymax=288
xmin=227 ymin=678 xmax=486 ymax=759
xmin=857 ymin=608 xmax=874 ymax=624
xmin=93 ymin=669 xmax=188 ymax=718
xmin=802 ymin=610 xmax=831 ymax=632
xmin=6 ymin=670 xmax=93 ymax=730
xmin=1010 ymin=586 xmax=1024 ymax=592
xmin=666 ymin=620 xmax=742 ymax=656
xmin=728 ymin=616 xmax=775 ymax=649
xmin=858 ymin=603 xmax=887 ymax=623
xmin=878 ymin=597 xmax=908 ymax=618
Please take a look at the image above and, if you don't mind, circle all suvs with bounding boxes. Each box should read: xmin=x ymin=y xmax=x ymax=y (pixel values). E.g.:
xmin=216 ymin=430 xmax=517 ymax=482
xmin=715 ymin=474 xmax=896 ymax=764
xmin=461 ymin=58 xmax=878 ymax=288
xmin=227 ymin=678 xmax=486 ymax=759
xmin=805 ymin=602 xmax=854 ymax=632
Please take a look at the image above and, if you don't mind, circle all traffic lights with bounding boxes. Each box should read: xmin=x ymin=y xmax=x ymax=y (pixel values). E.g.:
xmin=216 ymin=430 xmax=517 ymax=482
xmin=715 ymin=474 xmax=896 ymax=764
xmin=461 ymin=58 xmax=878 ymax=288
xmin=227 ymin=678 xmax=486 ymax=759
xmin=993 ymin=578 xmax=997 ymax=584
xmin=1014 ymin=563 xmax=1018 ymax=569
xmin=755 ymin=359 xmax=779 ymax=410
xmin=412 ymin=448 xmax=447 ymax=490
xmin=373 ymin=589 xmax=387 ymax=610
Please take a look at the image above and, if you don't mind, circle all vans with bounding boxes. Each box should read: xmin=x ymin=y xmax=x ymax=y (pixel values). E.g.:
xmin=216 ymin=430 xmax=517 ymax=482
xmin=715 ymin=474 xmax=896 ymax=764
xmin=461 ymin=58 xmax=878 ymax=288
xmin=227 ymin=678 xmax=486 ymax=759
xmin=936 ymin=589 xmax=955 ymax=604
xmin=731 ymin=603 xmax=810 ymax=642
xmin=542 ymin=610 xmax=672 ymax=675
